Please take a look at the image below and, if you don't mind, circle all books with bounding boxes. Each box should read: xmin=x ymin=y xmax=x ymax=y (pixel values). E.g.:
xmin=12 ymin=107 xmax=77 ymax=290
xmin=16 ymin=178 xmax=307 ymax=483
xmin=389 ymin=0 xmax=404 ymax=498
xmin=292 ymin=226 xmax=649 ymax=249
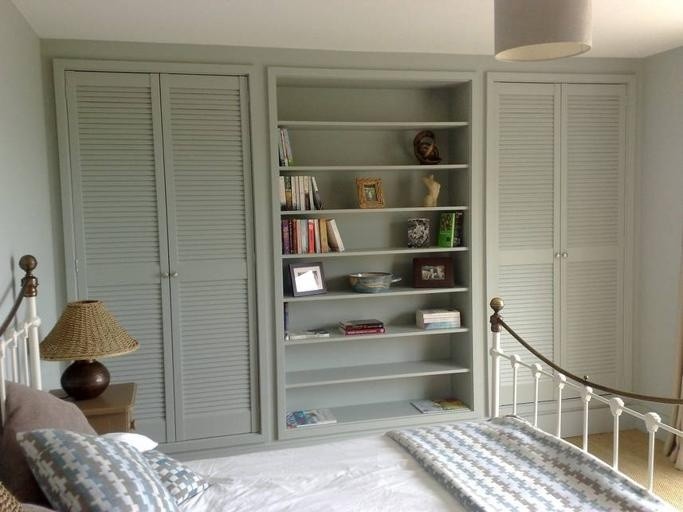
xmin=409 ymin=398 xmax=442 ymax=415
xmin=433 ymin=398 xmax=472 ymax=411
xmin=286 ymin=329 xmax=329 ymax=340
xmin=277 ymin=126 xmax=294 ymax=166
xmin=286 ymin=407 xmax=338 ymax=429
xmin=278 ymin=175 xmax=346 ymax=255
xmin=406 ymin=212 xmax=464 ymax=250
xmin=339 ymin=326 xmax=385 ymax=334
xmin=336 ymin=318 xmax=384 ymax=328
xmin=414 ymin=305 xmax=462 ymax=329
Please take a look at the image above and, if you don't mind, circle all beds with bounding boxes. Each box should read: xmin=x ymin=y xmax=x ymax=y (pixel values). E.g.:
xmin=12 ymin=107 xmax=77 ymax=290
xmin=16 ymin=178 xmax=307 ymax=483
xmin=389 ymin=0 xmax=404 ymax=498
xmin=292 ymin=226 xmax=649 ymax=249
xmin=1 ymin=253 xmax=682 ymax=512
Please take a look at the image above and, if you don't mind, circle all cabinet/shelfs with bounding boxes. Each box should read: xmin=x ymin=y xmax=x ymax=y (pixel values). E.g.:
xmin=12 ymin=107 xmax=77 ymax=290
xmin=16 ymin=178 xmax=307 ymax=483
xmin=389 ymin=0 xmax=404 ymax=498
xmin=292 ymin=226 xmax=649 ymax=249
xmin=262 ymin=51 xmax=483 ymax=442
xmin=42 ymin=42 xmax=273 ymax=453
xmin=482 ymin=57 xmax=640 ymax=439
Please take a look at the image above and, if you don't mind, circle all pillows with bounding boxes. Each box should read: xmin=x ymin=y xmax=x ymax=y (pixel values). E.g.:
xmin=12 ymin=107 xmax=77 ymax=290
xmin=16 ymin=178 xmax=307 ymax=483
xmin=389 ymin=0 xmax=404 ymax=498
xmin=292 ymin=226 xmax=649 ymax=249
xmin=1 ymin=379 xmax=210 ymax=511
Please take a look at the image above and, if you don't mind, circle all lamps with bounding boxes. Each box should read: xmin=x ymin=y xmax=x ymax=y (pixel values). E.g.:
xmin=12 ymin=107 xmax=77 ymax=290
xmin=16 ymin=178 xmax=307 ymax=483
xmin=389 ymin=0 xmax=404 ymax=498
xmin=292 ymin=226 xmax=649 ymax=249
xmin=38 ymin=297 xmax=141 ymax=402
xmin=493 ymin=0 xmax=592 ymax=62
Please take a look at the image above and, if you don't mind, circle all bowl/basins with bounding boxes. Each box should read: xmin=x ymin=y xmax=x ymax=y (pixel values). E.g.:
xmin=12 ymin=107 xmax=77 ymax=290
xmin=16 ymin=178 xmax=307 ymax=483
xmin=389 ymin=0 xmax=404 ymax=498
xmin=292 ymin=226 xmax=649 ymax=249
xmin=346 ymin=271 xmax=402 ymax=292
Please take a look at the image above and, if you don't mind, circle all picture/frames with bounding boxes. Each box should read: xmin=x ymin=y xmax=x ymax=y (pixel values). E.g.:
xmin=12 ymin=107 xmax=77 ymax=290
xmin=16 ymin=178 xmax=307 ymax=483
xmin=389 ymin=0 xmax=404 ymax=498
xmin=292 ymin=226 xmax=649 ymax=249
xmin=355 ymin=177 xmax=387 ymax=209
xmin=287 ymin=262 xmax=329 ymax=297
xmin=412 ymin=257 xmax=455 ymax=289
